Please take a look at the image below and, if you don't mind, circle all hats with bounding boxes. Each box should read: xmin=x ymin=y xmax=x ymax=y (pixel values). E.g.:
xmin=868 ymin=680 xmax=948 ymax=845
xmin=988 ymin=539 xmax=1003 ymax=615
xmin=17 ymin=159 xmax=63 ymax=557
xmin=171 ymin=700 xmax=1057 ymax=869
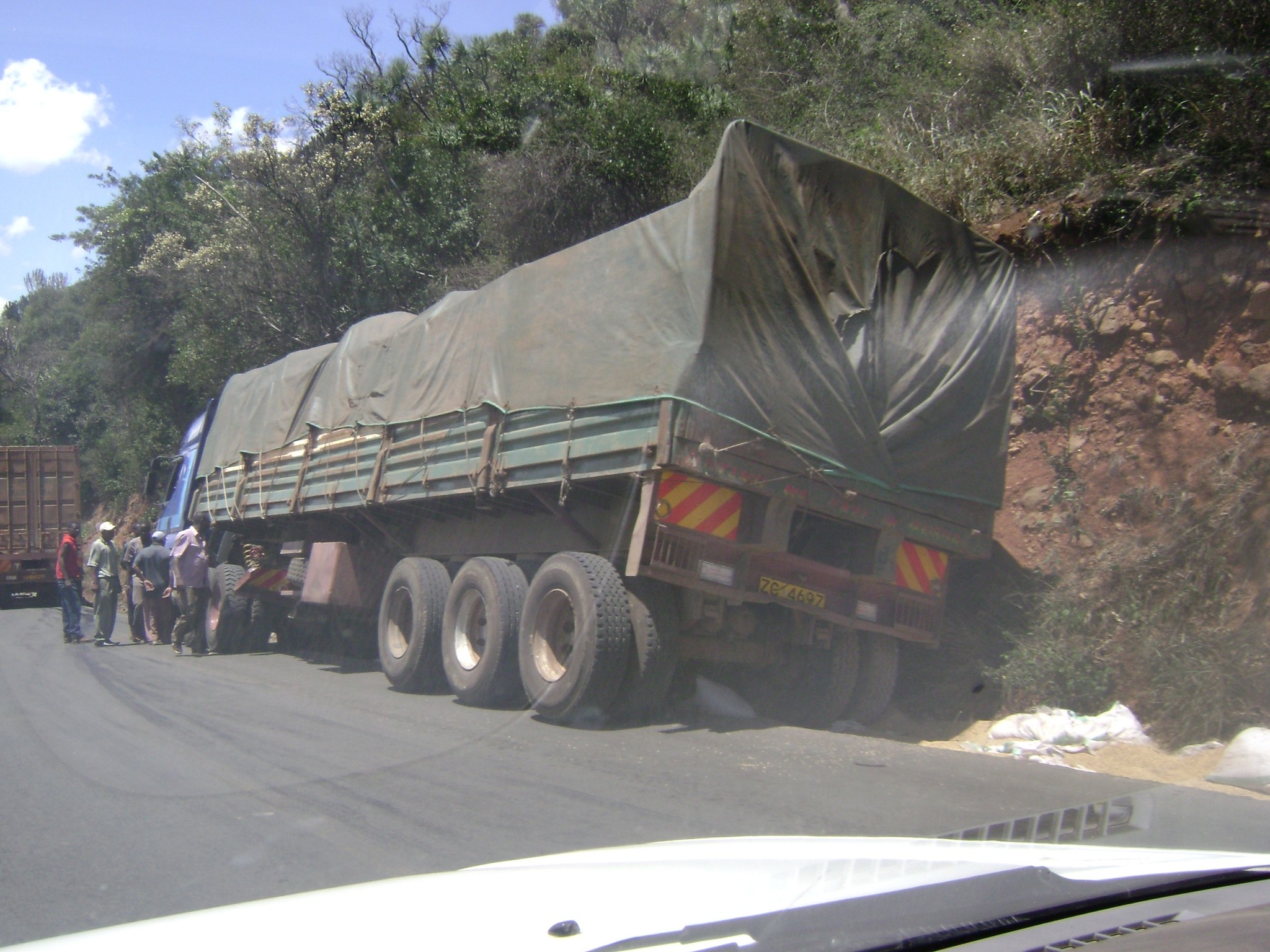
xmin=151 ymin=531 xmax=170 ymax=542
xmin=100 ymin=521 xmax=116 ymax=532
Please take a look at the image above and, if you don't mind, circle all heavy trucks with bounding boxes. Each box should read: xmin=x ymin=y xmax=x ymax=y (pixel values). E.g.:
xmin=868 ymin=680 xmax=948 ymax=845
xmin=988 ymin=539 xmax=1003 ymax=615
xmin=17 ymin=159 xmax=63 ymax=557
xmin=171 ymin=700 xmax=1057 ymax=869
xmin=142 ymin=119 xmax=1022 ymax=729
xmin=1 ymin=445 xmax=82 ymax=607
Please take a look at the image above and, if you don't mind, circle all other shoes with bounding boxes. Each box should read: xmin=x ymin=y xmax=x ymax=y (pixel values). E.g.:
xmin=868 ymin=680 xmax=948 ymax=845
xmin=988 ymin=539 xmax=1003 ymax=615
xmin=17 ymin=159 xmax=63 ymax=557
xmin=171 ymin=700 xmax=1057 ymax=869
xmin=192 ymin=648 xmax=210 ymax=656
xmin=171 ymin=639 xmax=182 ymax=654
xmin=64 ymin=637 xmax=72 ymax=643
xmin=104 ymin=640 xmax=119 ymax=646
xmin=153 ymin=639 xmax=163 ymax=645
xmin=208 ymin=650 xmax=218 ymax=655
xmin=72 ymin=638 xmax=91 ymax=643
xmin=132 ymin=634 xmax=141 ymax=642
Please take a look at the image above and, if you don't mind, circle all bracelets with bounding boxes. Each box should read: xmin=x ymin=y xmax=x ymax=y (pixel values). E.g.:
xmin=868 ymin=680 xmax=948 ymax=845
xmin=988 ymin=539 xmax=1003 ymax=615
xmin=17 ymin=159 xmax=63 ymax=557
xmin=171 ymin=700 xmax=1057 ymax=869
xmin=64 ymin=578 xmax=71 ymax=581
xmin=92 ymin=579 xmax=98 ymax=584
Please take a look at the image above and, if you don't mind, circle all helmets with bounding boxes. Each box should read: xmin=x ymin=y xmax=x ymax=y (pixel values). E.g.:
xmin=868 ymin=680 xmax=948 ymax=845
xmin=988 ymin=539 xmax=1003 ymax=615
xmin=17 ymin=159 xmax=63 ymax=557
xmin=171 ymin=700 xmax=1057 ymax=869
xmin=135 ymin=519 xmax=150 ymax=534
xmin=131 ymin=524 xmax=139 ymax=532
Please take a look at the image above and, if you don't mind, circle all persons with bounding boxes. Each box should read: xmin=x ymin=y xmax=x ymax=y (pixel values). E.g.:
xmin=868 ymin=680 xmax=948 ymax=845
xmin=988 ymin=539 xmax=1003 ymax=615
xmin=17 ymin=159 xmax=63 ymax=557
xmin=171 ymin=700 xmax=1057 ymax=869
xmin=56 ymin=521 xmax=93 ymax=644
xmin=169 ymin=511 xmax=218 ymax=657
xmin=87 ymin=521 xmax=123 ymax=647
xmin=120 ymin=521 xmax=175 ymax=645
